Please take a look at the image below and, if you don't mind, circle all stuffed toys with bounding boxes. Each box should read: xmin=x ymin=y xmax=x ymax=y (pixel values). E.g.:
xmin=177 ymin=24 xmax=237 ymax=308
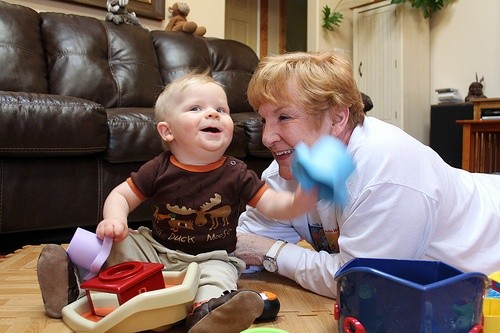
xmin=165 ymin=2 xmax=207 ymax=37
xmin=105 ymin=0 xmax=138 ymax=25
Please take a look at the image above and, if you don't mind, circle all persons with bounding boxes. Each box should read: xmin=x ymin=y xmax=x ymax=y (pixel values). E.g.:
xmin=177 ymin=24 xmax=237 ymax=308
xmin=38 ymin=68 xmax=319 ymax=333
xmin=236 ymin=51 xmax=500 ymax=301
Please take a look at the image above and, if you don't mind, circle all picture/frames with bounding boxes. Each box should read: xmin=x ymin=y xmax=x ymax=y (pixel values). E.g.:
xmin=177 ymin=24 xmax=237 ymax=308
xmin=60 ymin=0 xmax=165 ymax=20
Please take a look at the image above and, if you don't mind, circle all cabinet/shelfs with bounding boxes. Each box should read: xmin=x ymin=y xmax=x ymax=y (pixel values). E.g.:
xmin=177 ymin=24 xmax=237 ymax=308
xmin=350 ymin=0 xmax=431 ymax=149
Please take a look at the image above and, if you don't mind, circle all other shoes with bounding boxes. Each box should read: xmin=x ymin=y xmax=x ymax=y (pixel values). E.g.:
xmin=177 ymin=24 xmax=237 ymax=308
xmin=188 ymin=289 xmax=265 ymax=333
xmin=36 ymin=244 xmax=79 ymax=319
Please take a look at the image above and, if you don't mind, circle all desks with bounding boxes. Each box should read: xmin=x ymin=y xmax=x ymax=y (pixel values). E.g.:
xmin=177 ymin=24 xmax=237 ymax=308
xmin=456 ymin=98 xmax=500 ymax=174
xmin=430 ymin=102 xmax=491 ymax=173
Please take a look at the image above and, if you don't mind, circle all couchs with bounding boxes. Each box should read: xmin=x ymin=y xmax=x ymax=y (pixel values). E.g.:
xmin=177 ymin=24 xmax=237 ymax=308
xmin=0 ymin=0 xmax=372 ymax=250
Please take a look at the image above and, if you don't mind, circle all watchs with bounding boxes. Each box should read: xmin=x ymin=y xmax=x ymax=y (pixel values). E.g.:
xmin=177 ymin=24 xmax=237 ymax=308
xmin=262 ymin=239 xmax=286 ymax=274
